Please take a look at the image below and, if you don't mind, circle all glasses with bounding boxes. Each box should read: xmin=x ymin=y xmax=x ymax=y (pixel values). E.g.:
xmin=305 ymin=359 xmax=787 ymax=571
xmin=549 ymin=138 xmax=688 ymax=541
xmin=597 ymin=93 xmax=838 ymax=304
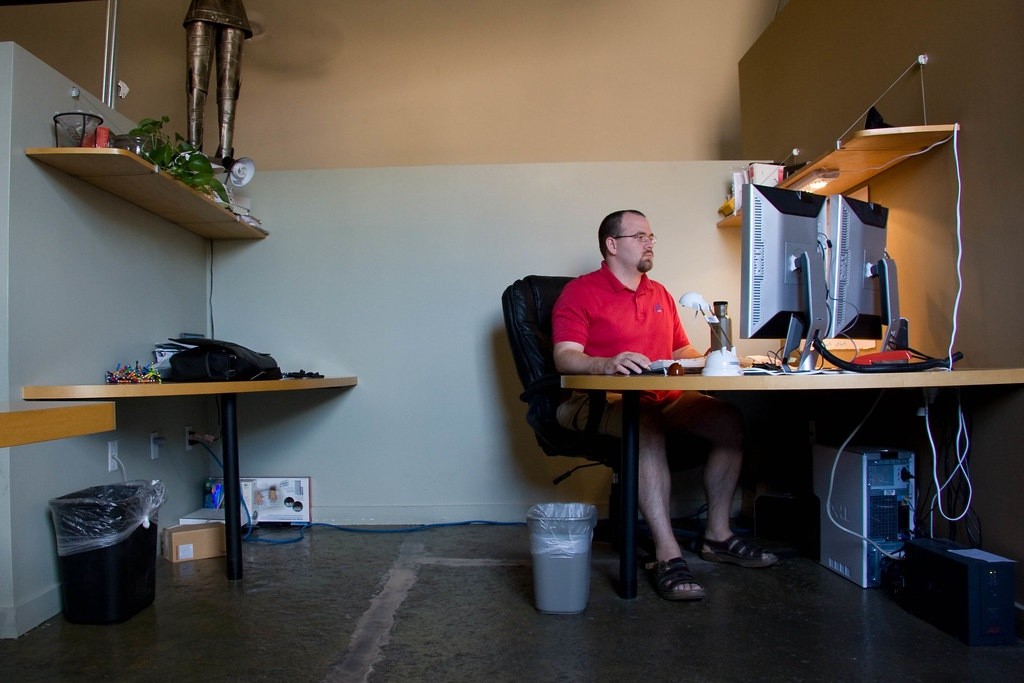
xmin=612 ymin=234 xmax=656 ymax=244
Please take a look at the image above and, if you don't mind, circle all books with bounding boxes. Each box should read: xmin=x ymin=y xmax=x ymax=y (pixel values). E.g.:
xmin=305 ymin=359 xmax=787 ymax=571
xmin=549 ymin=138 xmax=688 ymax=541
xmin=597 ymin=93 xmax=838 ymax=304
xmin=733 ymin=161 xmax=786 ymax=214
xmin=648 ymin=353 xmax=707 ymax=376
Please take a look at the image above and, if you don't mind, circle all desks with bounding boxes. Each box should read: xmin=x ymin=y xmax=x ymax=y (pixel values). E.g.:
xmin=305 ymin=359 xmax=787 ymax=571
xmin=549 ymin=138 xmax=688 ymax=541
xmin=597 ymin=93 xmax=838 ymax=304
xmin=560 ymin=368 xmax=1024 ymax=599
xmin=22 ymin=376 xmax=358 ymax=579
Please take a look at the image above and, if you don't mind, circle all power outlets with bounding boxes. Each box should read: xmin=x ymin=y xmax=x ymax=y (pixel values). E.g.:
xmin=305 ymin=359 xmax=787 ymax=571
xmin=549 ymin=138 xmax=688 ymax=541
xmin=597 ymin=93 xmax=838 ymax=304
xmin=185 ymin=427 xmax=192 ymax=451
xmin=150 ymin=432 xmax=159 ymax=460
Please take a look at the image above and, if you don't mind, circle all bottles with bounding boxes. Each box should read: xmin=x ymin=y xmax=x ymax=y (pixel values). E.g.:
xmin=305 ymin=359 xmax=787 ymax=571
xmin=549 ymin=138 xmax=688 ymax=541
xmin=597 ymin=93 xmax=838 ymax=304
xmin=710 ymin=301 xmax=733 ymax=351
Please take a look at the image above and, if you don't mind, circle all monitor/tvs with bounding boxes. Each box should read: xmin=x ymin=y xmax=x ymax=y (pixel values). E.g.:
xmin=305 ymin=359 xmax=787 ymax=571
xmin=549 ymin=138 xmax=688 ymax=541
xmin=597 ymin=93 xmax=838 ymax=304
xmin=827 ymin=195 xmax=914 ymax=366
xmin=739 ymin=183 xmax=857 ymax=375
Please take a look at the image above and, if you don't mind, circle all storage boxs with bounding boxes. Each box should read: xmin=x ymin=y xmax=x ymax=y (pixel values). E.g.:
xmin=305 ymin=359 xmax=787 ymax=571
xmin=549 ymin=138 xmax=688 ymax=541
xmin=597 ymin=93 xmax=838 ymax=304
xmin=179 ymin=508 xmax=225 ymax=526
xmin=205 ymin=479 xmax=258 ymax=525
xmin=160 ymin=523 xmax=225 ymax=564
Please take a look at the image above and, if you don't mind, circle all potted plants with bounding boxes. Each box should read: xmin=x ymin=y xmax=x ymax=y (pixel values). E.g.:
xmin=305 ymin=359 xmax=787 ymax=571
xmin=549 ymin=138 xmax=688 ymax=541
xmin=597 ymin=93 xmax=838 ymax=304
xmin=117 ymin=117 xmax=228 ymax=204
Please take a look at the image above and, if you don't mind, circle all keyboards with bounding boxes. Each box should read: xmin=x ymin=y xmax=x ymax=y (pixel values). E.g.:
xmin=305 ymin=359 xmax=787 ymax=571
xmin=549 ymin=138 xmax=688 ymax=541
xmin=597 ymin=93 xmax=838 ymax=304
xmin=649 ymin=357 xmax=707 ymax=373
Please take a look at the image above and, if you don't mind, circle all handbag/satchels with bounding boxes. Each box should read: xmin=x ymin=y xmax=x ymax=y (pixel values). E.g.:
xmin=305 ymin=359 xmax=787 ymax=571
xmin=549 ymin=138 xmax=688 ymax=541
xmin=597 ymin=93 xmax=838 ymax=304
xmin=169 ymin=337 xmax=282 ymax=381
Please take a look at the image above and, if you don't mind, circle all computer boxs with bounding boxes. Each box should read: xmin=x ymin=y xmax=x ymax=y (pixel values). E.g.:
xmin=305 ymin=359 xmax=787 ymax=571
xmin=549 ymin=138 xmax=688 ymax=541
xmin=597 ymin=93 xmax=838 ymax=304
xmin=813 ymin=444 xmax=917 ymax=589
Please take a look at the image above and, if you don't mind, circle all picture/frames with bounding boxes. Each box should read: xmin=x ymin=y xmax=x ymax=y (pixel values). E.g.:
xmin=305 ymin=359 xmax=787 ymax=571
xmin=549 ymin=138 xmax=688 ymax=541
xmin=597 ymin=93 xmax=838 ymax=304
xmin=209 ymin=476 xmax=312 ymax=528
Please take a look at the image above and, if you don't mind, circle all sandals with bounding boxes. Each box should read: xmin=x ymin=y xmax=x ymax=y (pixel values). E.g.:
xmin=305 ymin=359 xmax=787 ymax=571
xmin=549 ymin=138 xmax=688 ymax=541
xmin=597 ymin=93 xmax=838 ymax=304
xmin=656 ymin=557 xmax=706 ymax=600
xmin=701 ymin=535 xmax=778 ymax=567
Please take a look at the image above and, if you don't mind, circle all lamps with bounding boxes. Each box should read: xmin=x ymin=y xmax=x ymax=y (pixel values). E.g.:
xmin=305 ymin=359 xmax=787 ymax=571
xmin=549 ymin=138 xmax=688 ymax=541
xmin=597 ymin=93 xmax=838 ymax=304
xmin=217 ymin=146 xmax=255 ymax=192
xmin=790 ymin=167 xmax=841 ymax=205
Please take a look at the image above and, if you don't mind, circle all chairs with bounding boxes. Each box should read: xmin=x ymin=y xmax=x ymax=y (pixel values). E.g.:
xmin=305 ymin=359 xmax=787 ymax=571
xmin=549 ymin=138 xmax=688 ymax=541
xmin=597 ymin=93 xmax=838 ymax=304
xmin=502 ymin=274 xmax=702 ymax=552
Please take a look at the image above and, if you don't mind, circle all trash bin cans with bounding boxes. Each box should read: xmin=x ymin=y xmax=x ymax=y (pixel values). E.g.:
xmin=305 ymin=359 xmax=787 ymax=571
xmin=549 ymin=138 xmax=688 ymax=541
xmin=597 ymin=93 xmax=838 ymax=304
xmin=48 ymin=479 xmax=169 ymax=626
xmin=526 ymin=503 xmax=598 ymax=615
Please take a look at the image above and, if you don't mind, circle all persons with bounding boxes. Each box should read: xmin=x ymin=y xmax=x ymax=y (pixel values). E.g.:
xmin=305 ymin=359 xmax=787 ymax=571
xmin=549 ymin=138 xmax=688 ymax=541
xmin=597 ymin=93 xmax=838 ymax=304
xmin=181 ymin=0 xmax=253 ymax=178
xmin=550 ymin=209 xmax=779 ymax=599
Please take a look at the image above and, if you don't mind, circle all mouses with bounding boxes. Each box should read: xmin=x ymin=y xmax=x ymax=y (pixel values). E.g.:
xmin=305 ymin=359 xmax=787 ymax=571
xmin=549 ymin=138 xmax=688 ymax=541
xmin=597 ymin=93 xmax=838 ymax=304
xmin=617 ymin=361 xmax=649 ymax=375
